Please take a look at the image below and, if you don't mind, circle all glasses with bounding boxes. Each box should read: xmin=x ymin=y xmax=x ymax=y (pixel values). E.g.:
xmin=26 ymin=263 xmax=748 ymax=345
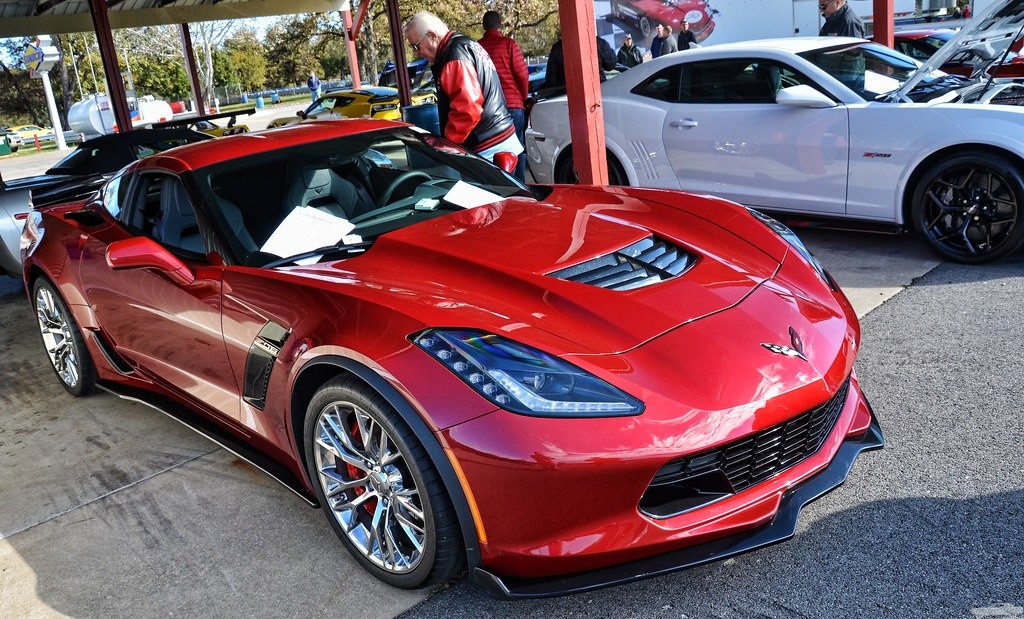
xmin=818 ymin=0 xmax=832 ymax=11
xmin=411 ymin=31 xmax=428 ymax=53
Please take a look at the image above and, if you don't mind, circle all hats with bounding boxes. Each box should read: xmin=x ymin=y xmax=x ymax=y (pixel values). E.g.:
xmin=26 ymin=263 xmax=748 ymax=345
xmin=624 ymin=34 xmax=632 ymax=39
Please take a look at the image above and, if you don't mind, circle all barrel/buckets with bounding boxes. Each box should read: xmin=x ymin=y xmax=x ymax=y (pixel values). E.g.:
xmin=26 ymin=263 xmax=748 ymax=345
xmin=255 ymin=96 xmax=264 ymax=108
xmin=242 ymin=94 xmax=248 ymax=103
xmin=271 ymin=92 xmax=279 ymax=103
xmin=401 ymin=103 xmax=441 ymax=169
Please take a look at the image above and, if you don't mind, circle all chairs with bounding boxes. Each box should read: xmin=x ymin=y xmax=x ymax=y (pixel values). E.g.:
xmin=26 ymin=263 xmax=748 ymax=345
xmin=152 ymin=175 xmax=259 ymax=262
xmin=755 ymin=63 xmax=784 ymax=104
xmin=282 ymin=162 xmax=376 ymax=221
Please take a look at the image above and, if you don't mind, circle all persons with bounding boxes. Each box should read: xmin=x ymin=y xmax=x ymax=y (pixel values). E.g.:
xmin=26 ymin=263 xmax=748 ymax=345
xmin=953 ymin=10 xmax=961 ymax=20
xmin=817 ymin=0 xmax=865 ymax=92
xmin=405 ymin=11 xmax=519 ymax=178
xmin=476 ymin=11 xmax=529 ymax=184
xmin=651 ymin=24 xmax=664 ymax=60
xmin=617 ymin=33 xmax=643 ymax=72
xmin=542 ymin=30 xmax=617 ymax=100
xmin=659 ymin=26 xmax=677 ymax=56
xmin=307 ymin=71 xmax=322 ymax=109
xmin=939 ymin=18 xmax=945 ymax=22
xmin=678 ymin=19 xmax=697 ymax=52
xmin=963 ymin=6 xmax=970 ymax=18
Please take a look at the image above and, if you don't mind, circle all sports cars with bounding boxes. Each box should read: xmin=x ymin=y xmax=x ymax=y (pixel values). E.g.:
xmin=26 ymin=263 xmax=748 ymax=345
xmin=14 ymin=118 xmax=885 ymax=600
xmin=0 ymin=108 xmax=257 ymax=278
xmin=267 ymin=59 xmax=438 ymax=130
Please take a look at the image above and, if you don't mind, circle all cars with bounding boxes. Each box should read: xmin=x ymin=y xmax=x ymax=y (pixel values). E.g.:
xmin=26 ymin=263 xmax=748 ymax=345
xmin=524 ymin=0 xmax=1024 ymax=265
xmin=0 ymin=124 xmax=55 ymax=152
